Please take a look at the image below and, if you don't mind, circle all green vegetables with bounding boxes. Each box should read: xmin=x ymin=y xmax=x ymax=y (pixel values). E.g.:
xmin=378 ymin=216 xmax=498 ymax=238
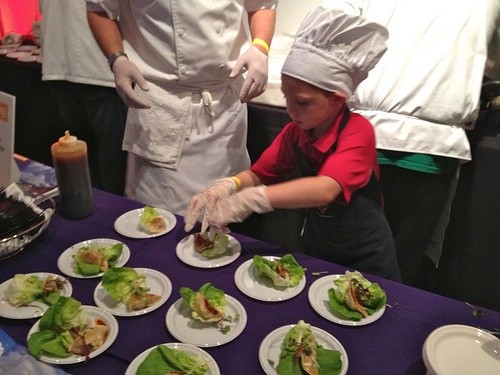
xmin=2 ymin=205 xmax=387 ymax=375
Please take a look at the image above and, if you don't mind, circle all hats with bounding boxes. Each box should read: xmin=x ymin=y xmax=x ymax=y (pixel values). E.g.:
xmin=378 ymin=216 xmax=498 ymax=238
xmin=280 ymin=0 xmax=390 ymax=99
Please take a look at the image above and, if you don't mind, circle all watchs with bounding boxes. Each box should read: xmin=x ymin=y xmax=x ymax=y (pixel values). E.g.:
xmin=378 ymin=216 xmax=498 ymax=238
xmin=108 ymin=50 xmax=128 ymax=73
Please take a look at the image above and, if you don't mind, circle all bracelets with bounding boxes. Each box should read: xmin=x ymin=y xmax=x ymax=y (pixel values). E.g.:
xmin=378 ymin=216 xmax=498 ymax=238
xmin=251 ymin=38 xmax=270 ymax=53
xmin=230 ymin=177 xmax=241 ymax=190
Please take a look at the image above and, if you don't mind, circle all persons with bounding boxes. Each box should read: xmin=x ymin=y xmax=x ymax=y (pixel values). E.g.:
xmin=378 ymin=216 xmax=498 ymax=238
xmin=183 ymin=3 xmax=406 ymax=284
xmin=37 ymin=0 xmax=277 ymax=234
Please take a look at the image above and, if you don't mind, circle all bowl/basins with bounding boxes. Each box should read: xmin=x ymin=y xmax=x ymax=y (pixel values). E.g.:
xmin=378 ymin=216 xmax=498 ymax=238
xmin=0 ymin=193 xmax=56 ymax=260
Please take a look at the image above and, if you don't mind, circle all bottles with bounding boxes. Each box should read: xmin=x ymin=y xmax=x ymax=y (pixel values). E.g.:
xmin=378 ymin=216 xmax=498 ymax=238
xmin=51 ymin=130 xmax=94 ymax=220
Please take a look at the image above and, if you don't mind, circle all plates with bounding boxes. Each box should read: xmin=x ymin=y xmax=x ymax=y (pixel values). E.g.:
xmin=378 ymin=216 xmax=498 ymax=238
xmin=175 ymin=232 xmax=241 ymax=268
xmin=259 ymin=324 xmax=349 ymax=375
xmin=124 ymin=343 xmax=220 ymax=375
xmin=0 ymin=272 xmax=72 ymax=319
xmin=57 ymin=238 xmax=130 ymax=278
xmin=27 ymin=305 xmax=118 ymax=364
xmin=422 ymin=324 xmax=500 ymax=375
xmin=0 ymin=44 xmax=42 ymax=62
xmin=94 ymin=268 xmax=172 ymax=316
xmin=114 ymin=208 xmax=177 ymax=239
xmin=165 ymin=292 xmax=247 ymax=348
xmin=308 ymin=274 xmax=385 ymax=326
xmin=234 ymin=256 xmax=306 ymax=302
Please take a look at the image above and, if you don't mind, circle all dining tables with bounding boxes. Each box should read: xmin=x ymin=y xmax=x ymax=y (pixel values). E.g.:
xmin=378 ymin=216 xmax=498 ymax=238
xmin=0 ymin=152 xmax=500 ymax=375
